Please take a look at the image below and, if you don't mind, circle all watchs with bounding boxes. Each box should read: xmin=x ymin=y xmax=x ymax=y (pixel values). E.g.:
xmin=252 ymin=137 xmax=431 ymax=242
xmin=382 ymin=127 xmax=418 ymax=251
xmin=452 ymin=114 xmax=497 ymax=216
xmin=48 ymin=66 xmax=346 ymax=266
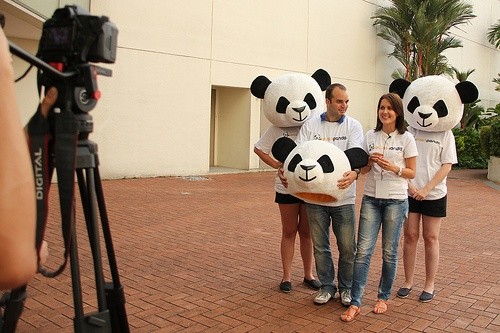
xmin=396 ymin=167 xmax=402 ymax=176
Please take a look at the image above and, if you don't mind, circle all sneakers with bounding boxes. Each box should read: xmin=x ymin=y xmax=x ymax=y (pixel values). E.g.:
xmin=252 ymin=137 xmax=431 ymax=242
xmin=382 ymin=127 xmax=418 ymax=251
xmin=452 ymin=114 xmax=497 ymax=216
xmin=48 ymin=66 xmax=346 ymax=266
xmin=313 ymin=290 xmax=332 ymax=304
xmin=340 ymin=290 xmax=353 ymax=306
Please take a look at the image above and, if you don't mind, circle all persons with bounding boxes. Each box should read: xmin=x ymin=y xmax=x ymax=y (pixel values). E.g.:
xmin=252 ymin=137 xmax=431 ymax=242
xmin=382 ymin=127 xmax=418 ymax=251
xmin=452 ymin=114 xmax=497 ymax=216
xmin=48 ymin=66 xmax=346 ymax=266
xmin=249 ymin=68 xmax=331 ymax=292
xmin=278 ymin=84 xmax=364 ymax=305
xmin=0 ymin=26 xmax=97 ymax=289
xmin=340 ymin=93 xmax=418 ymax=321
xmin=389 ymin=75 xmax=479 ymax=302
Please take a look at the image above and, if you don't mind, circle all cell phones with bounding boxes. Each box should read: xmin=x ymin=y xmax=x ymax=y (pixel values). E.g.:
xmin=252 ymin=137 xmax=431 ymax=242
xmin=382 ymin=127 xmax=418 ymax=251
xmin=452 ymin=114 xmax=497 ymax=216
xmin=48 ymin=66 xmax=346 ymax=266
xmin=0 ymin=121 xmax=130 ymax=333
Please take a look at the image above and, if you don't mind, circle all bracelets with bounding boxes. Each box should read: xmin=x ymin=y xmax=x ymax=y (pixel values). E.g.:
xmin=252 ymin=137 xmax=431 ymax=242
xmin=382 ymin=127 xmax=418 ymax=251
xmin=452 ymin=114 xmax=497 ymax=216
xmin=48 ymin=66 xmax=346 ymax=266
xmin=354 ymin=169 xmax=360 ymax=180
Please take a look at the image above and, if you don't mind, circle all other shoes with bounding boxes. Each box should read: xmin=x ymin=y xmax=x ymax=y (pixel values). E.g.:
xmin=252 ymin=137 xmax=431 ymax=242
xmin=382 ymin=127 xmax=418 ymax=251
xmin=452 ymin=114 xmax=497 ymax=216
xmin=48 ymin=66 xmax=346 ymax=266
xmin=280 ymin=276 xmax=292 ymax=293
xmin=303 ymin=276 xmax=322 ymax=290
xmin=419 ymin=289 xmax=435 ymax=303
xmin=396 ymin=285 xmax=413 ymax=299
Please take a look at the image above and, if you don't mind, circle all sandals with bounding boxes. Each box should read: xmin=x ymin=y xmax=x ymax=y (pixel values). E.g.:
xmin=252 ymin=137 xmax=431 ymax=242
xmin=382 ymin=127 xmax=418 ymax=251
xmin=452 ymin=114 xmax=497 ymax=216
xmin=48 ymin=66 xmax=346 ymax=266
xmin=340 ymin=305 xmax=360 ymax=322
xmin=372 ymin=297 xmax=388 ymax=314
